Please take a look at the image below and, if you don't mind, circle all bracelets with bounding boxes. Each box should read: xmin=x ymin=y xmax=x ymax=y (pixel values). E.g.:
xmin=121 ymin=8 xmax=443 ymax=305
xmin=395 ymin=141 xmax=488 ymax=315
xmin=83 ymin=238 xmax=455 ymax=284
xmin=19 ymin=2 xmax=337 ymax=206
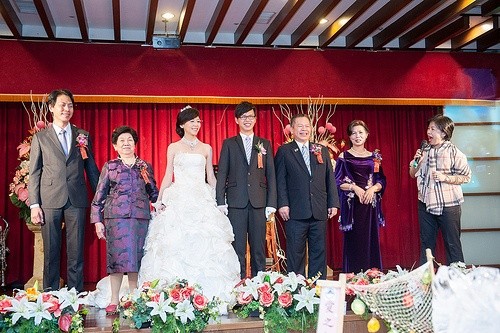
xmin=447 ymin=176 xmax=451 ymax=183
xmin=349 ymin=185 xmax=352 ymax=191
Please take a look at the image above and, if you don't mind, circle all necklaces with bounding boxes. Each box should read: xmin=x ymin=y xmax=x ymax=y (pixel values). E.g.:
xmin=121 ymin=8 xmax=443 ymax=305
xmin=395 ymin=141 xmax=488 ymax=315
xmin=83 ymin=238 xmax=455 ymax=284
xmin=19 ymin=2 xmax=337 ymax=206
xmin=351 ymin=147 xmax=366 ymax=154
xmin=182 ymin=137 xmax=199 ymax=146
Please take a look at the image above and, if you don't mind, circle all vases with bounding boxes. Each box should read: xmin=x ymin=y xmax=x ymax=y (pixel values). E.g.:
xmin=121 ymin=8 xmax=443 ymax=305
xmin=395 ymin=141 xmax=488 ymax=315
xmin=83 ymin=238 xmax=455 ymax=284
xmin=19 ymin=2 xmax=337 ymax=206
xmin=248 ymin=300 xmax=293 ymax=318
xmin=131 ymin=316 xmax=151 ymax=328
xmin=80 ymin=313 xmax=88 ymax=327
xmin=346 ymin=295 xmax=356 ymax=309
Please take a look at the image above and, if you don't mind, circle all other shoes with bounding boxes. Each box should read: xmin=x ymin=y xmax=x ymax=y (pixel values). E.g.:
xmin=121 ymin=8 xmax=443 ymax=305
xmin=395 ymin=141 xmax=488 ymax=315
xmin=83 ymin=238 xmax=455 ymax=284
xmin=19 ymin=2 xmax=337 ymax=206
xmin=106 ymin=301 xmax=120 ymax=312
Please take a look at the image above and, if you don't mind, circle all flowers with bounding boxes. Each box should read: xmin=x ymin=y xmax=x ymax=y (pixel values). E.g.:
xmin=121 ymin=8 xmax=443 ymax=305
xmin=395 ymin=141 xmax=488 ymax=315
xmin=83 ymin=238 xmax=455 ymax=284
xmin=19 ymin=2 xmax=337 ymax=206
xmin=113 ymin=277 xmax=221 ymax=333
xmin=231 ymin=267 xmax=319 ymax=333
xmin=76 ymin=133 xmax=88 ymax=147
xmin=270 ymin=92 xmax=343 ymax=169
xmin=373 ymin=148 xmax=382 ymax=163
xmin=343 ymin=270 xmax=429 ymax=333
xmin=134 ymin=160 xmax=147 ymax=169
xmin=309 ymin=144 xmax=324 ymax=156
xmin=255 ymin=141 xmax=269 ymax=157
xmin=9 ymin=85 xmax=57 ymax=220
xmin=0 ymin=278 xmax=90 ymax=333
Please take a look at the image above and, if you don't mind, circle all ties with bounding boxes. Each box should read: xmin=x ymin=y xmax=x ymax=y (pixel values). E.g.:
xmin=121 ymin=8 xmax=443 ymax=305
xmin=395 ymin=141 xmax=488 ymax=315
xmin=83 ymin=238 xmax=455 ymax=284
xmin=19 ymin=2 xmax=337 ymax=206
xmin=59 ymin=130 xmax=69 ymax=157
xmin=244 ymin=137 xmax=252 ymax=165
xmin=302 ymin=146 xmax=312 ymax=176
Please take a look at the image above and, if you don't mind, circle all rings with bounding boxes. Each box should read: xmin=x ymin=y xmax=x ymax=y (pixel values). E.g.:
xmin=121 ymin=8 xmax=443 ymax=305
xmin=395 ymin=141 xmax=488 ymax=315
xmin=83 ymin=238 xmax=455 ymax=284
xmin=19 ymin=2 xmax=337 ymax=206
xmin=436 ymin=175 xmax=437 ymax=178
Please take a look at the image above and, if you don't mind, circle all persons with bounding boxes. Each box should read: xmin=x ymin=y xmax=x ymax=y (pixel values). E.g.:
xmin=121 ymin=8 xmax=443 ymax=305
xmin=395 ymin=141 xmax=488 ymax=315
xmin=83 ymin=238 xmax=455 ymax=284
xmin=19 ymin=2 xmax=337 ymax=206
xmin=335 ymin=120 xmax=386 ymax=274
xmin=29 ymin=90 xmax=101 ymax=295
xmin=274 ymin=114 xmax=339 ymax=279
xmin=90 ymin=126 xmax=159 ymax=314
xmin=137 ymin=106 xmax=241 ymax=302
xmin=409 ymin=114 xmax=471 ymax=267
xmin=216 ymin=102 xmax=277 ymax=279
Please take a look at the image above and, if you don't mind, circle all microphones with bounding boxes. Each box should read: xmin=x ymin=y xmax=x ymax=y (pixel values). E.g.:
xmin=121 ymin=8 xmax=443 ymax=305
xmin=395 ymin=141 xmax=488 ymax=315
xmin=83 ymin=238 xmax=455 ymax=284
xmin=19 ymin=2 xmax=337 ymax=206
xmin=413 ymin=140 xmax=427 ymax=168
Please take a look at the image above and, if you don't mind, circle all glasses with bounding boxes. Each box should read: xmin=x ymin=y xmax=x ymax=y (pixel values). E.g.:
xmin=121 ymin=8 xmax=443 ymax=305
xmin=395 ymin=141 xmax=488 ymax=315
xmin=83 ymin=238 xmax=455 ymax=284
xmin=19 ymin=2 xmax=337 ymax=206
xmin=237 ymin=114 xmax=256 ymax=120
